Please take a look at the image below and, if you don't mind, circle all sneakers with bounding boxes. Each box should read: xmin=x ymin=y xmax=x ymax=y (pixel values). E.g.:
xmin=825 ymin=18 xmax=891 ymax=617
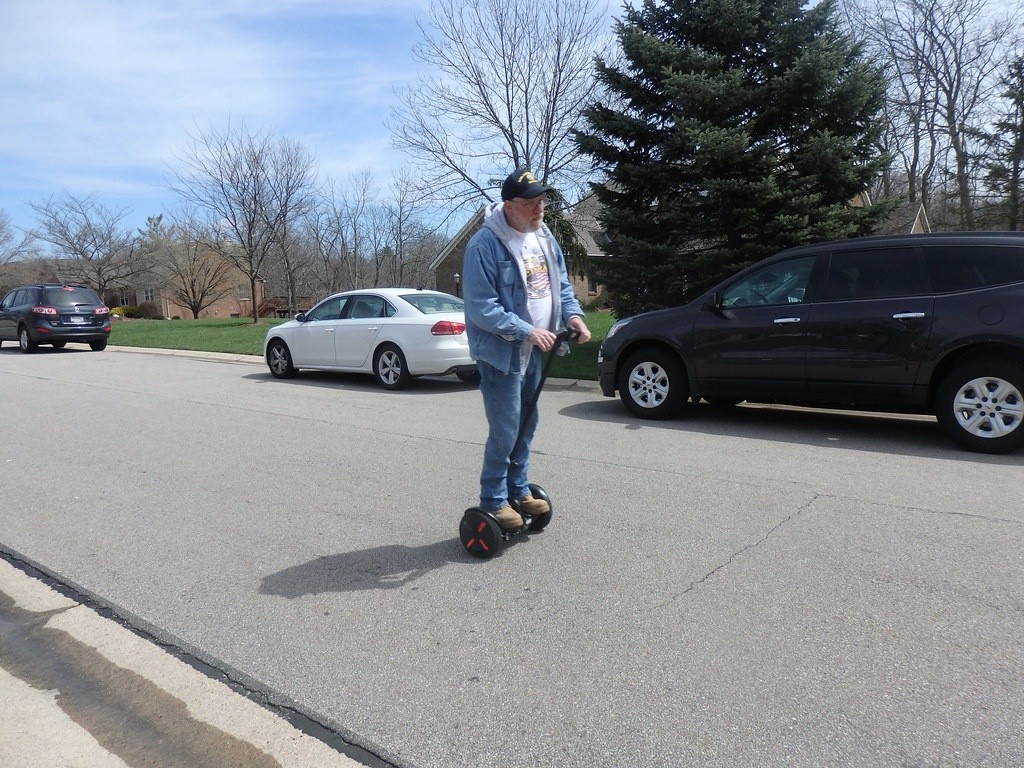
xmin=492 ymin=494 xmax=549 ymax=528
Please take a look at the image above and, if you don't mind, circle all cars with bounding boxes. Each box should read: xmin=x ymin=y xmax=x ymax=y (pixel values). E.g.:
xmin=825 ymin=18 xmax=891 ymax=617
xmin=264 ymin=285 xmax=480 ymax=389
xmin=0 ymin=281 xmax=110 ymax=352
xmin=597 ymin=220 xmax=1024 ymax=458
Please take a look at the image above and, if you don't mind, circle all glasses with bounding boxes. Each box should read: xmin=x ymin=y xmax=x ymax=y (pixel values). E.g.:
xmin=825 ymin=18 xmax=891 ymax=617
xmin=508 ymin=196 xmax=549 ymax=209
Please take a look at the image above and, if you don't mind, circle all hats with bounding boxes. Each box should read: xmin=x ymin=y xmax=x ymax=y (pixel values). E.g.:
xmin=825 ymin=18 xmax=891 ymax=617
xmin=500 ymin=170 xmax=553 ymax=202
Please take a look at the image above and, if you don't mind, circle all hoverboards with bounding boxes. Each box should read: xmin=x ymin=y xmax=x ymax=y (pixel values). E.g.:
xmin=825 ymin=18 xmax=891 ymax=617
xmin=459 ymin=328 xmax=585 ymax=558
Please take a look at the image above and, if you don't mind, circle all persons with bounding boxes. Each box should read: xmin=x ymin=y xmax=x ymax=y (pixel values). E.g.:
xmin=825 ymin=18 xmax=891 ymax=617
xmin=461 ymin=169 xmax=591 ymax=530
